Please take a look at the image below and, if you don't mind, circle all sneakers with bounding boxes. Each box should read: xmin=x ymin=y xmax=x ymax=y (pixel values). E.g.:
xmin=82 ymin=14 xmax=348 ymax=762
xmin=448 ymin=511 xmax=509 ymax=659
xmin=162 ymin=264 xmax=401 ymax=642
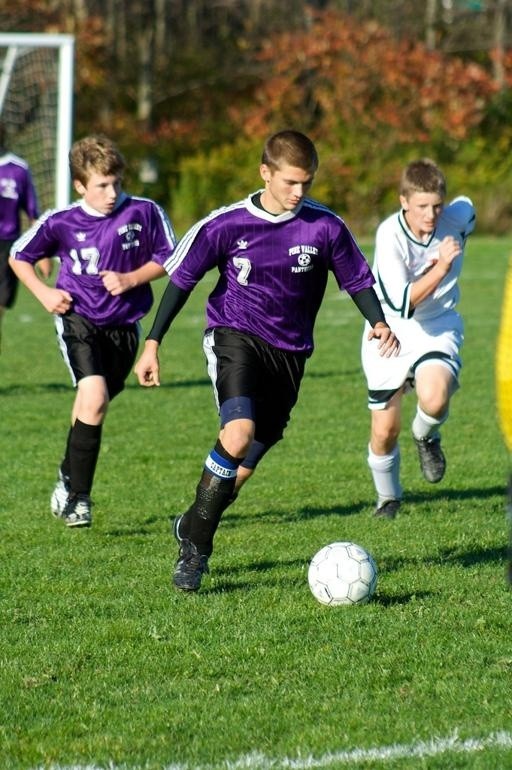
xmin=372 ymin=499 xmax=401 ymax=519
xmin=412 ymin=433 xmax=446 ymax=483
xmin=51 ymin=469 xmax=95 ymax=527
xmin=172 ymin=511 xmax=210 ymax=592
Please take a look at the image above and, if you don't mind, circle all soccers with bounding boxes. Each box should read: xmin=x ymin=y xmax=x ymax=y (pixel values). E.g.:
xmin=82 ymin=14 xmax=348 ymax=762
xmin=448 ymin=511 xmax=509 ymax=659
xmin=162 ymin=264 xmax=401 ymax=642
xmin=308 ymin=541 xmax=379 ymax=606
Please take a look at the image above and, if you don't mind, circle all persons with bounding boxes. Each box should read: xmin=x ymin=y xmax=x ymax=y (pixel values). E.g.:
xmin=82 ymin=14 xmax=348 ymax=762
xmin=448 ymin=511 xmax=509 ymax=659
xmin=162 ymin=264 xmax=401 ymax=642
xmin=0 ymin=137 xmax=53 ymax=312
xmin=133 ymin=131 xmax=401 ymax=591
xmin=361 ymin=161 xmax=476 ymax=522
xmin=7 ymin=135 xmax=178 ymax=526
